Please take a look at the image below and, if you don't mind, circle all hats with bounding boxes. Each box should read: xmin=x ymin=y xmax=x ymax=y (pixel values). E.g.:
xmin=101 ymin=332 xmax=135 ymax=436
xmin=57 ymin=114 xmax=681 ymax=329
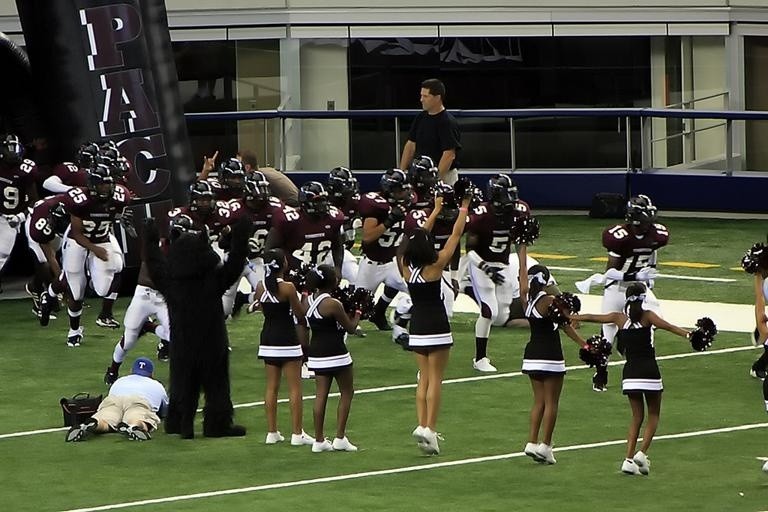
xmin=161 ymin=227 xmax=220 ymax=279
xmin=131 ymin=359 xmax=155 ymax=380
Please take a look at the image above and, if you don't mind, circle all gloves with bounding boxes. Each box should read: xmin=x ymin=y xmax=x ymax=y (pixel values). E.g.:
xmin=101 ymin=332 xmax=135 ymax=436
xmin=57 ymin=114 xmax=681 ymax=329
xmin=4 ymin=212 xmax=24 ymax=231
xmin=115 ymin=206 xmax=141 ymax=239
xmin=636 ymin=266 xmax=660 ymax=282
xmin=478 ymin=260 xmax=507 ymax=287
xmin=649 ymin=279 xmax=656 ymax=290
xmin=383 ymin=206 xmax=409 ymax=229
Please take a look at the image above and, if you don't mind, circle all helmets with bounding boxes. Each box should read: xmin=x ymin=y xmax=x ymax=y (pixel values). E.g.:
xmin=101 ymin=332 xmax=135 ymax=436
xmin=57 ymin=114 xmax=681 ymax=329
xmin=488 ymin=174 xmax=514 ymax=204
xmin=47 ymin=201 xmax=70 ymax=236
xmin=74 ymin=140 xmax=127 ymax=197
xmin=327 ymin=166 xmax=359 ymax=198
xmin=242 ymin=170 xmax=271 ymax=203
xmin=413 ymin=154 xmax=439 ymax=178
xmin=378 ymin=167 xmax=412 ymax=196
xmin=1 ymin=132 xmax=27 ymax=168
xmin=220 ymin=156 xmax=248 ymax=179
xmin=625 ymin=194 xmax=656 ymax=223
xmin=189 ymin=179 xmax=217 ymax=212
xmin=428 ymin=183 xmax=457 ymax=211
xmin=298 ymin=179 xmax=330 ymax=215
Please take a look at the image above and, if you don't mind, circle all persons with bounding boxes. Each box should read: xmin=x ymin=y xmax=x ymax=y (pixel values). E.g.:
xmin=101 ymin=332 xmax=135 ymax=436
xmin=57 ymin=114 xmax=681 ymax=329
xmin=433 ymin=245 xmax=472 ymax=322
xmin=67 ymin=358 xmax=168 ymax=443
xmin=24 ymin=140 xmax=138 ymax=347
xmin=473 ymin=243 xmax=524 ymax=371
xmin=105 ymin=215 xmax=193 ymax=386
xmin=400 ymin=193 xmax=473 ymax=457
xmin=266 ymin=216 xmax=342 ymax=296
xmin=548 ymin=283 xmax=718 ymax=475
xmin=247 ymin=248 xmax=316 ymax=445
xmin=340 ymin=217 xmax=400 ymax=331
xmin=480 ymin=173 xmax=518 ymax=192
xmin=525 ymin=243 xmax=560 ymax=326
xmin=741 ymin=243 xmax=768 ymax=471
xmin=401 ymin=192 xmax=540 ymax=243
xmin=522 ymin=265 xmax=613 ymax=463
xmin=301 ymin=264 xmax=375 ymax=452
xmin=168 ymin=152 xmax=400 ymax=217
xmin=143 ymin=216 xmax=257 ymax=440
xmin=392 ymin=274 xmax=415 ymax=348
xmin=575 ymin=195 xmax=669 ymax=392
xmin=400 ymin=79 xmax=481 ymax=192
xmin=0 ymin=136 xmax=43 ymax=271
xmin=193 ymin=216 xmax=266 ymax=319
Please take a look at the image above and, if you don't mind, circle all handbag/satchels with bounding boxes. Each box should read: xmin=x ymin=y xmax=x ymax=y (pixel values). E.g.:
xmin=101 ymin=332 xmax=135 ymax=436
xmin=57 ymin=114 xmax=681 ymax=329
xmin=60 ymin=393 xmax=102 ymax=429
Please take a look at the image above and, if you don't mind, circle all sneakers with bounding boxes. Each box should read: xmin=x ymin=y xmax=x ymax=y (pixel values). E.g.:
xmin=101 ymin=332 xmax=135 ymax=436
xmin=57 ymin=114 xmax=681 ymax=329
xmin=333 ymin=436 xmax=359 ymax=453
xmin=750 ymin=364 xmax=764 ymax=378
xmin=634 ymin=451 xmax=650 ymax=475
xmin=593 ymin=372 xmax=608 ymax=393
xmin=64 ymin=416 xmax=99 ymax=444
xmin=472 ymin=356 xmax=499 ymax=374
xmin=156 ymin=342 xmax=172 ymax=364
xmin=24 ymin=276 xmax=58 ymax=328
xmin=66 ymin=325 xmax=87 ymax=348
xmin=535 ymin=441 xmax=557 ymax=467
xmin=95 ymin=313 xmax=122 ymax=331
xmin=103 ymin=365 xmax=119 ymax=386
xmin=523 ymin=443 xmax=546 ymax=462
xmin=204 ymin=424 xmax=248 ymax=438
xmin=397 ymin=332 xmax=413 ymax=352
xmin=368 ymin=314 xmax=393 ymax=332
xmin=247 ymin=298 xmax=260 ymax=313
xmin=621 ymin=459 xmax=644 ymax=479
xmin=117 ymin=420 xmax=154 ymax=441
xmin=290 ymin=433 xmax=318 ymax=448
xmin=414 ymin=425 xmax=444 ymax=457
xmin=311 ymin=440 xmax=335 ymax=455
xmin=264 ymin=431 xmax=286 ymax=445
